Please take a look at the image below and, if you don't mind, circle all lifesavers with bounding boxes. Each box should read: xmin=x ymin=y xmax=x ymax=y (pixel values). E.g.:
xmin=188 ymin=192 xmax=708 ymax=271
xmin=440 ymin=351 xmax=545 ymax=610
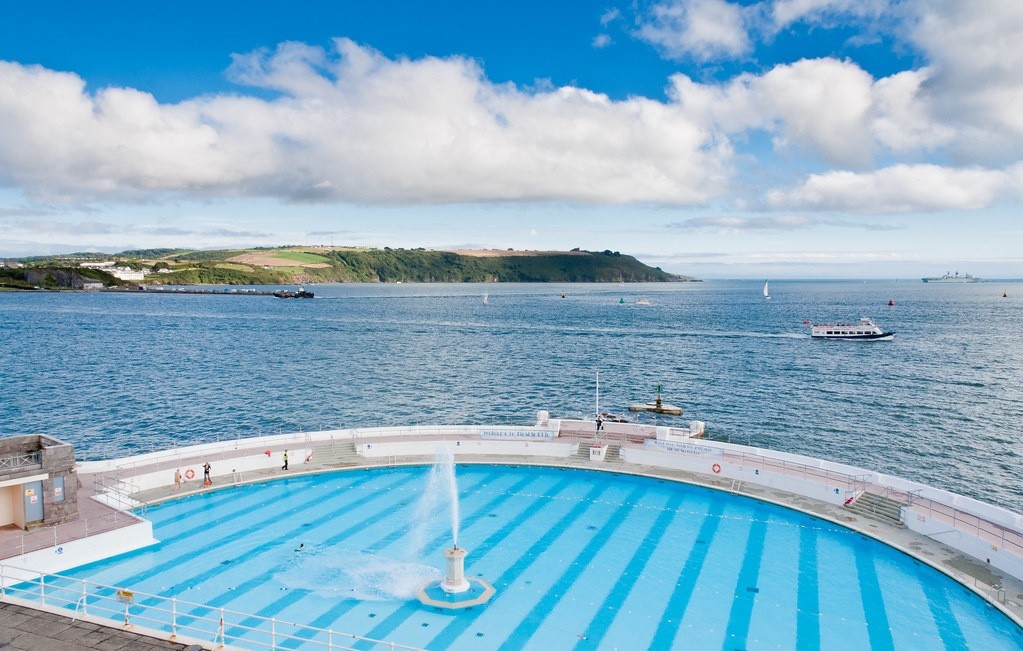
xmin=186 ymin=469 xmax=195 ymax=479
xmin=712 ymin=463 xmax=722 ymax=473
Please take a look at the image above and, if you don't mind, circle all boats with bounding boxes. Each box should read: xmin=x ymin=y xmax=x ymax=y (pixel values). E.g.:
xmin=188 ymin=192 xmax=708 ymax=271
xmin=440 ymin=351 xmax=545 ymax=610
xmin=272 ymin=288 xmax=315 ymax=298
xmin=922 ymin=272 xmax=980 ymax=284
xmin=808 ymin=317 xmax=895 ymax=341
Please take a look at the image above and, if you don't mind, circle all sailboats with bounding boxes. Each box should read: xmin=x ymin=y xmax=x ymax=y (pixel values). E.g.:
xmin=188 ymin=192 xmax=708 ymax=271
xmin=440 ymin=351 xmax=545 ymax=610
xmin=762 ymin=279 xmax=771 ymax=301
